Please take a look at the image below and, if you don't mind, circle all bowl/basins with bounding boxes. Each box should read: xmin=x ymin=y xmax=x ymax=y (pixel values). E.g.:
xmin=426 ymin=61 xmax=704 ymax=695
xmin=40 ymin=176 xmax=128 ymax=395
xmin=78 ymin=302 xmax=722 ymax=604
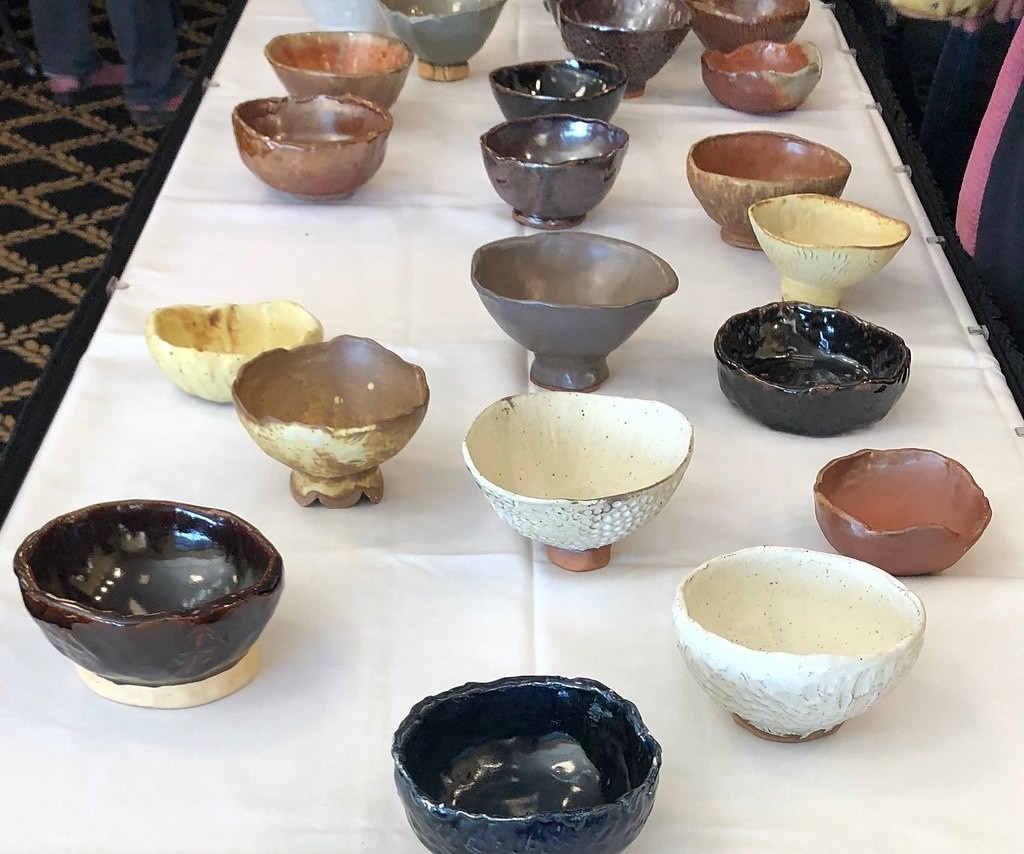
xmin=264 ymin=30 xmax=414 ymax=107
xmin=701 ymin=39 xmax=822 ymax=115
xmin=489 ymin=58 xmax=628 ymax=121
xmin=686 ymin=130 xmax=851 ymax=250
xmin=231 ymin=333 xmax=430 ymax=508
xmin=748 ymin=193 xmax=911 ymax=308
xmin=672 ymin=545 xmax=926 ymax=742
xmin=392 ymin=674 xmax=662 ymax=854
xmin=480 ymin=114 xmax=630 ymax=230
xmin=145 ymin=301 xmax=324 ymax=403
xmin=297 ymin=0 xmax=507 ymax=82
xmin=12 ymin=499 xmax=284 ymax=709
xmin=813 ymin=448 xmax=993 ymax=575
xmin=714 ymin=300 xmax=912 ymax=438
xmin=543 ymin=0 xmax=690 ymax=98
xmin=232 ymin=92 xmax=394 ymax=204
xmin=462 ymin=391 xmax=694 ymax=572
xmin=685 ymin=0 xmax=811 ymax=52
xmin=470 ymin=230 xmax=679 ymax=392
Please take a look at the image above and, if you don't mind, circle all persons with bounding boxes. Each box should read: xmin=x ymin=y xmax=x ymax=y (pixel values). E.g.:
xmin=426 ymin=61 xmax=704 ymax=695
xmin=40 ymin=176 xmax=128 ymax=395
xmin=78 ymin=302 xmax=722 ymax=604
xmin=914 ymin=0 xmax=1024 ymax=372
xmin=27 ymin=0 xmax=197 ymax=128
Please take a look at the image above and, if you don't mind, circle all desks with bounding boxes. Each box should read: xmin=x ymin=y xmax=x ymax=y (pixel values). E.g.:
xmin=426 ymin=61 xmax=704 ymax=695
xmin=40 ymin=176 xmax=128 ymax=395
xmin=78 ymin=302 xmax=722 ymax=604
xmin=1 ymin=0 xmax=1024 ymax=854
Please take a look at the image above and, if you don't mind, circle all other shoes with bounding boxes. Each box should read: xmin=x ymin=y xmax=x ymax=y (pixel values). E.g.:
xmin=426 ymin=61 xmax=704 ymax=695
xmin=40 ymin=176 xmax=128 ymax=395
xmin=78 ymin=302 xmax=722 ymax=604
xmin=130 ymin=80 xmax=192 ymax=124
xmin=50 ymin=59 xmax=125 ymax=106
